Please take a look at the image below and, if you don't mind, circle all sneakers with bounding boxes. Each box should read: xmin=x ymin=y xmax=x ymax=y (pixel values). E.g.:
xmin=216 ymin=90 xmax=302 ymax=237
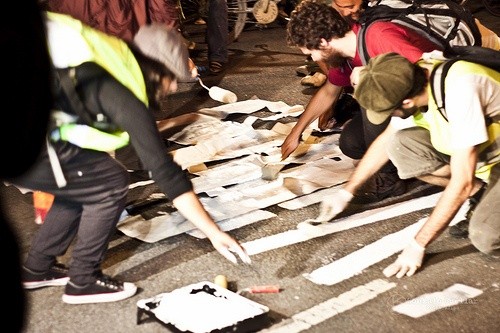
xmin=21 ymin=262 xmax=70 ymax=289
xmin=62 ymin=274 xmax=138 ymax=305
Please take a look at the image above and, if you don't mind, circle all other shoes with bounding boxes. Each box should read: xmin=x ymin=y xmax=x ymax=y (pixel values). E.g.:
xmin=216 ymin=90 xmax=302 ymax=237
xmin=296 ymin=64 xmax=311 ymax=75
xmin=208 ymin=60 xmax=226 ymax=73
xmin=301 ymin=70 xmax=327 ymax=86
xmin=449 ymin=199 xmax=478 ymax=238
xmin=351 ymin=166 xmax=405 ymax=203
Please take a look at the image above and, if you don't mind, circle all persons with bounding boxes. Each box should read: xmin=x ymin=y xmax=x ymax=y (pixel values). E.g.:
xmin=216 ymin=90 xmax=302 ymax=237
xmin=205 ymin=0 xmax=500 ymax=162
xmin=3 ymin=11 xmax=251 ymax=305
xmin=0 ymin=0 xmax=57 ymax=333
xmin=315 ymin=52 xmax=500 ymax=279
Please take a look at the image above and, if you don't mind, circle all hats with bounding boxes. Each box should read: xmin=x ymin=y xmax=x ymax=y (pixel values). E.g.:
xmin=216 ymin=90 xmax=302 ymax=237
xmin=134 ymin=24 xmax=192 ymax=82
xmin=353 ymin=51 xmax=414 ymax=124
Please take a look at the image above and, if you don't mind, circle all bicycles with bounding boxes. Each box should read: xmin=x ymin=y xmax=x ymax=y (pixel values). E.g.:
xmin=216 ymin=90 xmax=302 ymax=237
xmin=172 ymin=0 xmax=303 ymax=52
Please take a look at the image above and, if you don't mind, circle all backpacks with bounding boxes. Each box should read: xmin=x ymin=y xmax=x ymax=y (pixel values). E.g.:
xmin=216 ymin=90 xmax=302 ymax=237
xmin=429 ymin=43 xmax=500 ymax=127
xmin=356 ymin=0 xmax=482 ymax=65
xmin=41 ymin=11 xmax=149 ymax=188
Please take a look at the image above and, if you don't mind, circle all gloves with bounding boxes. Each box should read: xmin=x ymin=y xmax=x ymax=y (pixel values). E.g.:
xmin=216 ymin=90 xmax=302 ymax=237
xmin=383 ymin=237 xmax=426 ymax=278
xmin=317 ymin=189 xmax=354 ymax=224
